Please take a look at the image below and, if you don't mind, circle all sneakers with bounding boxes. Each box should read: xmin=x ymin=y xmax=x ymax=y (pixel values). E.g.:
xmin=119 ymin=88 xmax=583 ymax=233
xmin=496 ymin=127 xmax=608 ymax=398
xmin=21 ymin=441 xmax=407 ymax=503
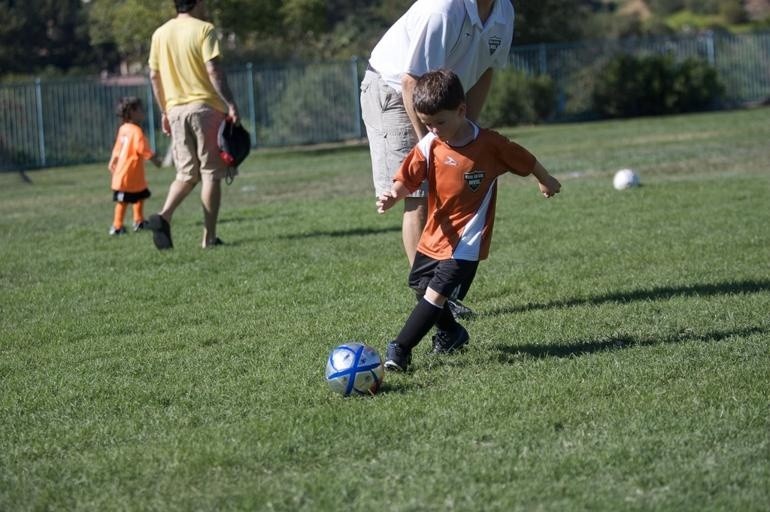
xmin=448 ymin=299 xmax=472 ymax=319
xmin=429 ymin=324 xmax=470 ymax=354
xmin=149 ymin=214 xmax=172 ymax=249
xmin=109 ymin=226 xmax=128 ymax=235
xmin=133 ymin=221 xmax=149 ymax=231
xmin=384 ymin=341 xmax=411 ymax=373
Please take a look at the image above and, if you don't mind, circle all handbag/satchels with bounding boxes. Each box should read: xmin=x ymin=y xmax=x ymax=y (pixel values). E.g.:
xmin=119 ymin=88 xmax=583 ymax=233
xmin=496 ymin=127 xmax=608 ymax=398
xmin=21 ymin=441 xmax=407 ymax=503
xmin=217 ymin=116 xmax=250 ymax=167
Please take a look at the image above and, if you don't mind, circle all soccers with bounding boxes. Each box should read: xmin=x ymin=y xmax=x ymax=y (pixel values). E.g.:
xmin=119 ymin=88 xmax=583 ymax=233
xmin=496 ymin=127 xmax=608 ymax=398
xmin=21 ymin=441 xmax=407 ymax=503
xmin=613 ymin=169 xmax=638 ymax=191
xmin=325 ymin=342 xmax=384 ymax=397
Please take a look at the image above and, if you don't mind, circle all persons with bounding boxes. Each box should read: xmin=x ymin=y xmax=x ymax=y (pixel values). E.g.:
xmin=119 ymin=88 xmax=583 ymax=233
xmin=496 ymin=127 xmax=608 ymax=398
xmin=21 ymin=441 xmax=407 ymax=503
xmin=359 ymin=0 xmax=515 ymax=320
xmin=148 ymin=0 xmax=242 ymax=250
xmin=375 ymin=69 xmax=561 ymax=374
xmin=108 ymin=96 xmax=161 ymax=237
